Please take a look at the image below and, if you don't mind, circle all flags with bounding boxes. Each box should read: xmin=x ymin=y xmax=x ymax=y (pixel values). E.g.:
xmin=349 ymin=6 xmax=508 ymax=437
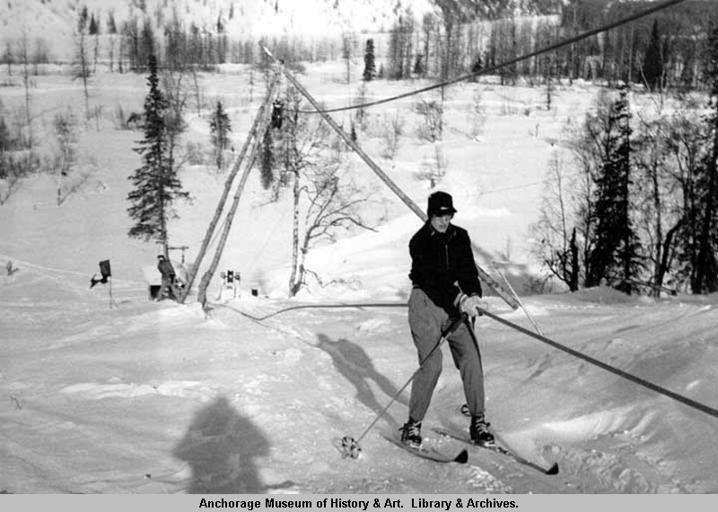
xmin=99 ymin=260 xmax=110 ymax=276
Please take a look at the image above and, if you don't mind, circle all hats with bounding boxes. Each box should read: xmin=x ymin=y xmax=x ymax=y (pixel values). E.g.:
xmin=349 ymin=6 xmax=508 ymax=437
xmin=426 ymin=190 xmax=457 ymax=218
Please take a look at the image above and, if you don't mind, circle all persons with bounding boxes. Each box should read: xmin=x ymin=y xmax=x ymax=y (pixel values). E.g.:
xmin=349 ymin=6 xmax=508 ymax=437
xmin=153 ymin=254 xmax=176 ymax=302
xmin=400 ymin=190 xmax=499 ymax=449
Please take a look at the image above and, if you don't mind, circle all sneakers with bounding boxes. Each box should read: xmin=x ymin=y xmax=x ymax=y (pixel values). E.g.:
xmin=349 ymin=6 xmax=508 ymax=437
xmin=401 ymin=421 xmax=423 ymax=449
xmin=469 ymin=421 xmax=494 ymax=445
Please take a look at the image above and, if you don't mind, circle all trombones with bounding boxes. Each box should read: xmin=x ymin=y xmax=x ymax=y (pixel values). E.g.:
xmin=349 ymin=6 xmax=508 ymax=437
xmin=376 ymin=425 xmax=560 ymax=474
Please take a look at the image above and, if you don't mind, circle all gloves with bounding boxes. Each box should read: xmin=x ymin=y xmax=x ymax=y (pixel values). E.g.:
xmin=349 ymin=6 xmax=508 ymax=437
xmin=458 ymin=295 xmax=488 ymax=317
